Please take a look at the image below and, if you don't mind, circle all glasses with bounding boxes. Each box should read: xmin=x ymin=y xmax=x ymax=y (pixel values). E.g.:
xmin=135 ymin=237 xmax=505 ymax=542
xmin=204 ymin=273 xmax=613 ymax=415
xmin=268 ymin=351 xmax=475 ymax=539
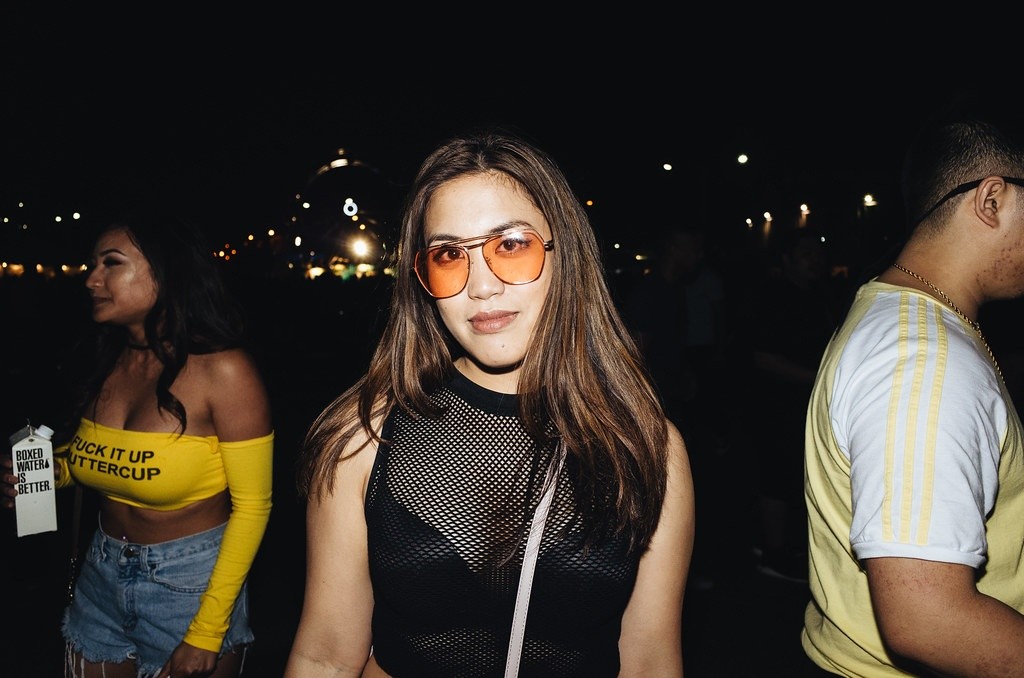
xmin=412 ymin=226 xmax=558 ymax=299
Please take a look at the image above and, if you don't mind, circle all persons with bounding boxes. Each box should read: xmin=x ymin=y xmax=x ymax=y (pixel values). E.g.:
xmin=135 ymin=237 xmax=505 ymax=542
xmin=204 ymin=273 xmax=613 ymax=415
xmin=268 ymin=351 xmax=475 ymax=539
xmin=284 ymin=134 xmax=695 ymax=678
xmin=798 ymin=93 xmax=1024 ymax=678
xmin=0 ymin=206 xmax=277 ymax=678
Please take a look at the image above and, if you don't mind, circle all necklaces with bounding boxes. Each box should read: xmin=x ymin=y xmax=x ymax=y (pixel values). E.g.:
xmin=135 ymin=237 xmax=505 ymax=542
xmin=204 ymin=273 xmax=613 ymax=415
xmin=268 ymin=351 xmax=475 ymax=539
xmin=894 ymin=264 xmax=1006 ymax=384
